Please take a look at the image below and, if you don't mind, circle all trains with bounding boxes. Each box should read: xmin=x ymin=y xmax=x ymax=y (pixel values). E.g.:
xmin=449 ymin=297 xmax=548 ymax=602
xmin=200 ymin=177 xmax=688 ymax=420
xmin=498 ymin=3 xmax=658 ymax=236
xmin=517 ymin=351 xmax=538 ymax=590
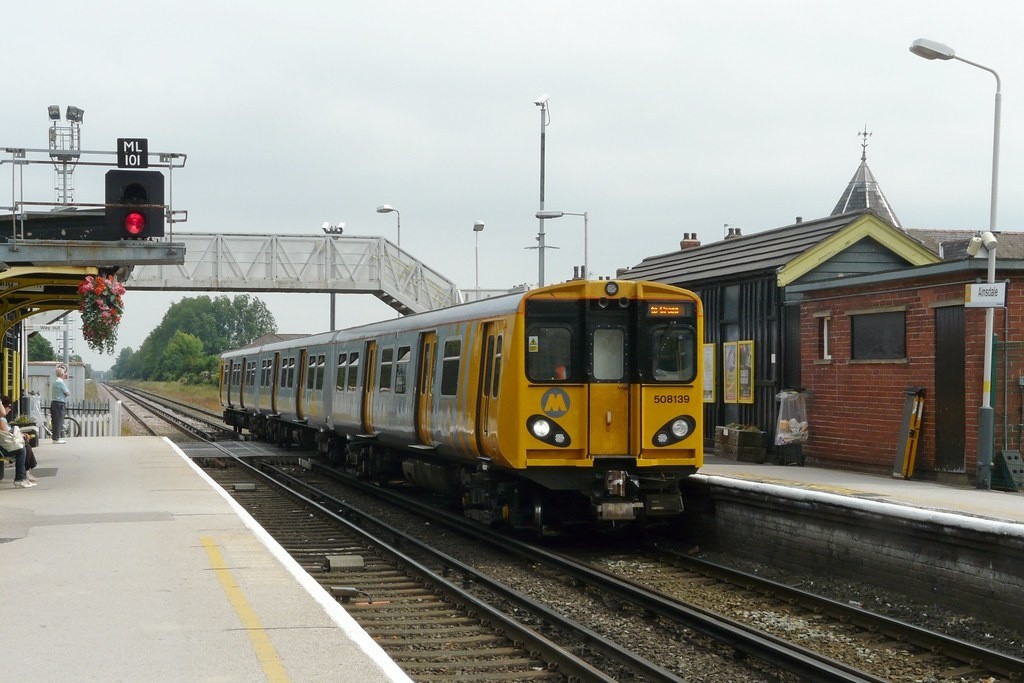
xmin=214 ymin=273 xmax=707 ymax=540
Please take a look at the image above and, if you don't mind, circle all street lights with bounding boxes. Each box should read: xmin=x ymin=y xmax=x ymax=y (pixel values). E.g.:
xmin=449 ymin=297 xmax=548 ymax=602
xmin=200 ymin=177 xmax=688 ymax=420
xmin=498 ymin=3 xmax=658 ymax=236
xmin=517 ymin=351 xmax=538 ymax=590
xmin=321 ymin=220 xmax=348 ymax=331
xmin=471 ymin=220 xmax=484 ymax=301
xmin=533 ymin=208 xmax=591 ymax=279
xmin=374 ymin=203 xmax=402 ymax=281
xmin=907 ymin=36 xmax=1002 ymax=492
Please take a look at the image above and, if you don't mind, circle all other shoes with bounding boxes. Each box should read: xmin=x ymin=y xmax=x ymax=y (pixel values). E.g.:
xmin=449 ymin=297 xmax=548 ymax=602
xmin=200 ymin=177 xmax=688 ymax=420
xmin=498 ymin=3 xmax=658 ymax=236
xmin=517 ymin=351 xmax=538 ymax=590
xmin=26 ymin=472 xmax=37 ymax=481
xmin=53 ymin=439 xmax=66 ymax=444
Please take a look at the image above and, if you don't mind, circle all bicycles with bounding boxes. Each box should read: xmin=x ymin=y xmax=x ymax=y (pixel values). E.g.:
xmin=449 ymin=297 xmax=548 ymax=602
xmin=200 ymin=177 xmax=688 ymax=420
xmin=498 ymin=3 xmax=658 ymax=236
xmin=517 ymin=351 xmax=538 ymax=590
xmin=27 ymin=389 xmax=81 ymax=439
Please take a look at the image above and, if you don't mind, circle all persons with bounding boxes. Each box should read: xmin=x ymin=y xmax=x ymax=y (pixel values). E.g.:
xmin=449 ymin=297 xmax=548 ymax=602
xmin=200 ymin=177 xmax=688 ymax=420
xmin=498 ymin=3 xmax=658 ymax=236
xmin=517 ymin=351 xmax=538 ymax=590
xmin=50 ymin=368 xmax=71 ymax=444
xmin=0 ymin=394 xmax=39 ymax=488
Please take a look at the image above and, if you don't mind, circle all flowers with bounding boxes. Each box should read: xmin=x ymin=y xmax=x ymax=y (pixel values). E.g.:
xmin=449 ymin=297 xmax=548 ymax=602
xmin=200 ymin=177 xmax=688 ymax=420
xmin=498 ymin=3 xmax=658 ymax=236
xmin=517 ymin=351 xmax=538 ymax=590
xmin=76 ymin=276 xmax=126 ymax=357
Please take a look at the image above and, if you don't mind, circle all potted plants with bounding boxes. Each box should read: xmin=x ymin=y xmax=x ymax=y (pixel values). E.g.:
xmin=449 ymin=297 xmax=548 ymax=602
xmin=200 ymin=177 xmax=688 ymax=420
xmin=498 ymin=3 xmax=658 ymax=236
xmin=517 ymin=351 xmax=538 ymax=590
xmin=8 ymin=415 xmax=38 ymax=448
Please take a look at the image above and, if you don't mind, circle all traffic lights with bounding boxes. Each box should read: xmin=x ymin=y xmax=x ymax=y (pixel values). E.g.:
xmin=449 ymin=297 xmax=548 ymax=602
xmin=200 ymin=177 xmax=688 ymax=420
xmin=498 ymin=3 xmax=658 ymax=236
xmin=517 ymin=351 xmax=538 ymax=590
xmin=102 ymin=168 xmax=165 ymax=240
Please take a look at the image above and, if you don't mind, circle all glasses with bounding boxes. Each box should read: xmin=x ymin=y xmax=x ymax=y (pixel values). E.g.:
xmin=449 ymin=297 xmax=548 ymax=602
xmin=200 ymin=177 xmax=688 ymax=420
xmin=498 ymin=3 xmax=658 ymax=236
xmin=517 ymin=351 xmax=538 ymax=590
xmin=6 ymin=406 xmax=12 ymax=409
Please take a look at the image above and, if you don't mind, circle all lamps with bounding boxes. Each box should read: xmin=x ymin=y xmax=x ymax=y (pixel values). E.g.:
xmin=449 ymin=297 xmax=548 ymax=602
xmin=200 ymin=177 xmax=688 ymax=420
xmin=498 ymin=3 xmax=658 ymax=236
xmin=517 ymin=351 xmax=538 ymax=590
xmin=967 ymin=230 xmax=999 ymax=256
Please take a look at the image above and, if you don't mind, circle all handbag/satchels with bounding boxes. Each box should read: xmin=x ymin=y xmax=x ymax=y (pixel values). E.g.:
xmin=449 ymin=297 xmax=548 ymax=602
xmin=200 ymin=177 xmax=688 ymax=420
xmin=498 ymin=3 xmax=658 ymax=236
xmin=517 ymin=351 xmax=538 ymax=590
xmin=0 ymin=426 xmax=24 ymax=452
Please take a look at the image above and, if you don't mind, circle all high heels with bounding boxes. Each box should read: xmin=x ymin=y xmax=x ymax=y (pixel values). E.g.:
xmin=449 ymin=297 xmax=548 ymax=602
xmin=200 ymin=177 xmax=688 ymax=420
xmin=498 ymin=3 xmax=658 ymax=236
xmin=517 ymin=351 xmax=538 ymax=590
xmin=15 ymin=479 xmax=36 ymax=488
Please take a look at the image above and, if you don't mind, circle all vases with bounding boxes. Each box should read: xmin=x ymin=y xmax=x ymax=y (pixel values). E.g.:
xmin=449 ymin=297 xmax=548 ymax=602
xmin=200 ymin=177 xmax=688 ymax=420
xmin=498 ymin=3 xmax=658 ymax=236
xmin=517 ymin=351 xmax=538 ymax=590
xmin=715 ymin=426 xmax=767 ymax=462
